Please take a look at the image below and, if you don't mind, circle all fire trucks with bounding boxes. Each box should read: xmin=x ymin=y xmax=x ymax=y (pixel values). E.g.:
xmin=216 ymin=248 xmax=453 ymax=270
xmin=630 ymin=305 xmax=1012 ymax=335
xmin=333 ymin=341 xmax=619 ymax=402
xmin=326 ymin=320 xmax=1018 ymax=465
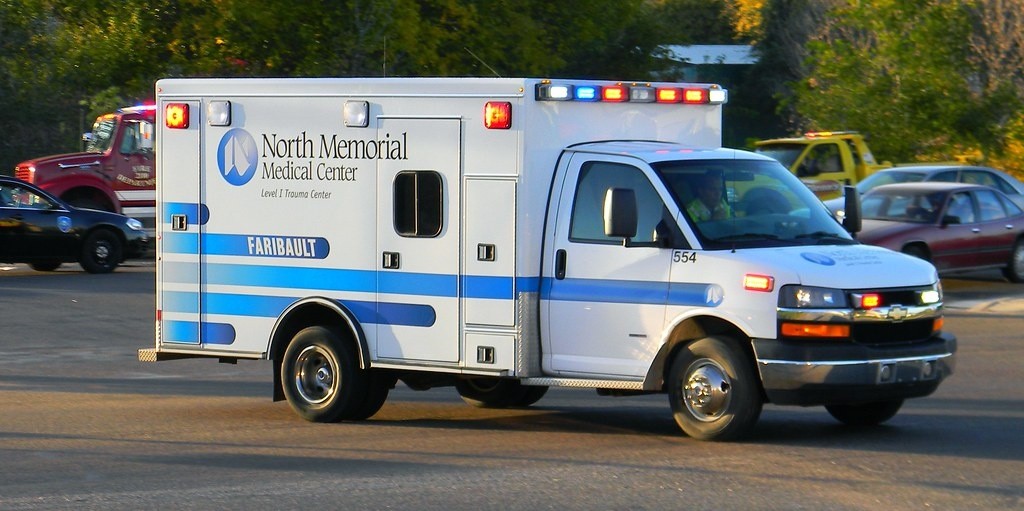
xmin=12 ymin=100 xmax=158 ymax=258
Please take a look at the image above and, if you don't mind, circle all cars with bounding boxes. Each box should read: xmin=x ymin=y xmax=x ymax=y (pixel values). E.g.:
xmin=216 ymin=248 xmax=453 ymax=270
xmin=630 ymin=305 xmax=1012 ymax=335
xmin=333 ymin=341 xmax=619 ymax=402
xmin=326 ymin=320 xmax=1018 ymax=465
xmin=835 ymin=182 xmax=1024 ymax=284
xmin=782 ymin=166 xmax=1024 ymax=235
xmin=0 ymin=175 xmax=152 ymax=274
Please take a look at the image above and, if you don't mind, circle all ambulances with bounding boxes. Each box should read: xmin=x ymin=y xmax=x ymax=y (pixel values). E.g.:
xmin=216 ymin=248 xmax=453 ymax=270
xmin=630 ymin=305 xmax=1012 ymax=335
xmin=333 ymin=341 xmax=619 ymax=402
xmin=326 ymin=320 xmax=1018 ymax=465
xmin=139 ymin=76 xmax=955 ymax=442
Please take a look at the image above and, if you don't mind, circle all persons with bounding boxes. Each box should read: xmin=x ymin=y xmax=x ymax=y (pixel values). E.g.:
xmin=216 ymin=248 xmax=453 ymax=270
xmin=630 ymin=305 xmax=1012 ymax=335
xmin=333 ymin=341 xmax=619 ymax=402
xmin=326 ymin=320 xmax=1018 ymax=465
xmin=685 ymin=171 xmax=749 ymax=223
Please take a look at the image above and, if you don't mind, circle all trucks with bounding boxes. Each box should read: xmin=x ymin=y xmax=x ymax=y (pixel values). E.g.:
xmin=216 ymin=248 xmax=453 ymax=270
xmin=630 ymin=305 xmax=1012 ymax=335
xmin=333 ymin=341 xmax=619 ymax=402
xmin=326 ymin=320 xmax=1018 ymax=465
xmin=687 ymin=130 xmax=998 ymax=212
